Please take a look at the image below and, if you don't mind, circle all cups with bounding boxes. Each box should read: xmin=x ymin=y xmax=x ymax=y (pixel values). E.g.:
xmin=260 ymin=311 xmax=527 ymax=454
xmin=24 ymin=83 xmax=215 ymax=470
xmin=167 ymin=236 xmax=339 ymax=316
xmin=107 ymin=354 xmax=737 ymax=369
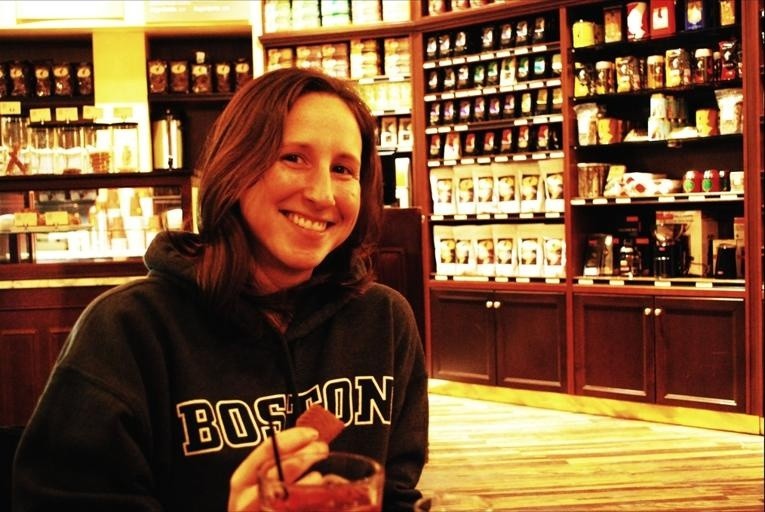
xmin=257 ymin=452 xmax=384 ymax=512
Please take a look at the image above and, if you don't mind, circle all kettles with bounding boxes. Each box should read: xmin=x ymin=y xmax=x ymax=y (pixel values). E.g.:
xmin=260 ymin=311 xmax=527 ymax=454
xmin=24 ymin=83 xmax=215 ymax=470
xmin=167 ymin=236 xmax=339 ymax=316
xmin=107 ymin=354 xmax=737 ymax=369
xmin=152 ymin=107 xmax=185 ymax=175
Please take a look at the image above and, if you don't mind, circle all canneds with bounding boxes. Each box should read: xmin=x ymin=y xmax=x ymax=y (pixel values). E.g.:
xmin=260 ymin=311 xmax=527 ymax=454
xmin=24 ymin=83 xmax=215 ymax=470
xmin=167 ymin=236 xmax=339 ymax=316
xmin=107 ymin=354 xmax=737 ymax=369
xmin=648 ymin=93 xmax=688 ymax=140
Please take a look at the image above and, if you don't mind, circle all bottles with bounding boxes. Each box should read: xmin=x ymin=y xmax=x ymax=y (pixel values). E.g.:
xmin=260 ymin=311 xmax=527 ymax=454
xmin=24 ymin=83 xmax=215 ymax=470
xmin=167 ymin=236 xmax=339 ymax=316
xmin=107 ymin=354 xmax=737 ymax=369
xmin=1 ymin=113 xmax=139 ymax=175
xmin=575 ymin=44 xmax=738 ymax=95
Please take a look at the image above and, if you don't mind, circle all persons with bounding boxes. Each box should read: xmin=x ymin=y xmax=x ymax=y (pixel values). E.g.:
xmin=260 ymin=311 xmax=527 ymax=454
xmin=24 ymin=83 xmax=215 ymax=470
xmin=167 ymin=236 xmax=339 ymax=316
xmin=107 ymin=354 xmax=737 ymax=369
xmin=11 ymin=68 xmax=436 ymax=512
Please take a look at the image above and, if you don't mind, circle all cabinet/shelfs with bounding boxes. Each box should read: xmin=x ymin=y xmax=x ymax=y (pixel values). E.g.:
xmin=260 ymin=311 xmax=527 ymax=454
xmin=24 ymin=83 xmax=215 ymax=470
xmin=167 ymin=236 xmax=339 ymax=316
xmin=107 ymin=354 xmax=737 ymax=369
xmin=411 ymin=0 xmax=763 ymax=435
xmin=1 ymin=0 xmax=413 ymax=274
